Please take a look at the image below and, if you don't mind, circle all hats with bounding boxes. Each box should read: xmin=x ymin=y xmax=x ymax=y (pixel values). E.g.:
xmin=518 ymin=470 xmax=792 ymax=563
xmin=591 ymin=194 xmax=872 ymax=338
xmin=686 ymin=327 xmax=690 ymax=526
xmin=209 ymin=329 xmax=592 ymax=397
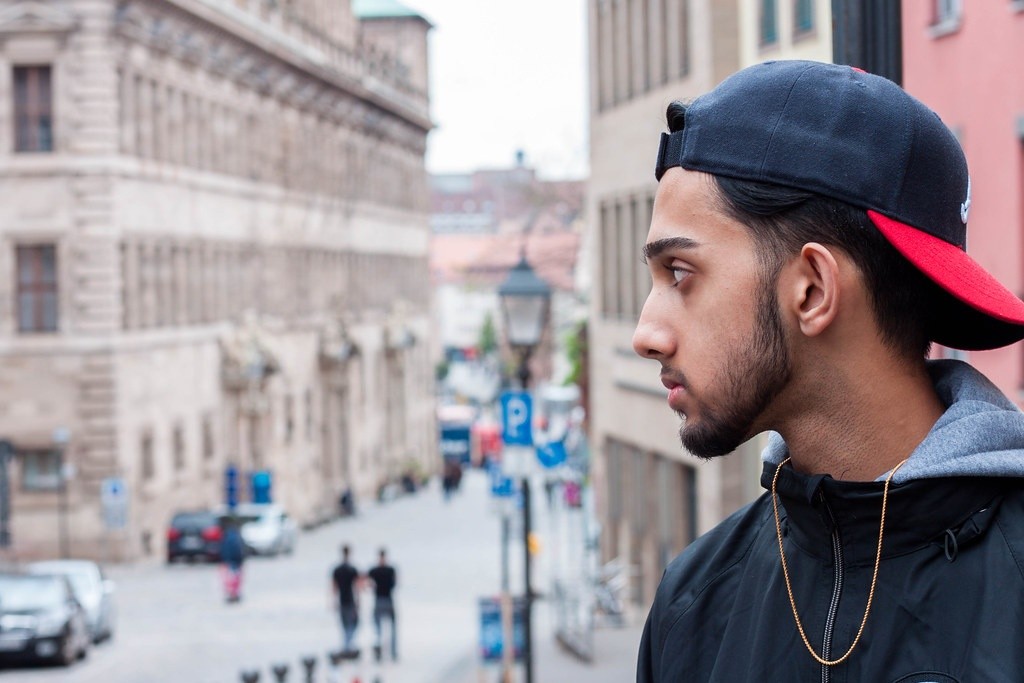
xmin=654 ymin=57 xmax=1024 ymax=353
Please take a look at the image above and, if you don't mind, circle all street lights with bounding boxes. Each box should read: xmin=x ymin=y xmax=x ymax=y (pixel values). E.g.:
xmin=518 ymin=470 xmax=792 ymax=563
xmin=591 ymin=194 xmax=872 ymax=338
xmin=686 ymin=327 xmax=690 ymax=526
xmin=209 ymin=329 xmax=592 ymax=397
xmin=498 ymin=243 xmax=553 ymax=683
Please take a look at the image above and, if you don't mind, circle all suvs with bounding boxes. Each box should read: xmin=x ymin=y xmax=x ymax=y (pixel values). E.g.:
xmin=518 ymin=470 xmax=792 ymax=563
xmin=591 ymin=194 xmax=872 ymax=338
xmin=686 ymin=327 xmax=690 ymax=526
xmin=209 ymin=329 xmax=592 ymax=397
xmin=167 ymin=502 xmax=302 ymax=561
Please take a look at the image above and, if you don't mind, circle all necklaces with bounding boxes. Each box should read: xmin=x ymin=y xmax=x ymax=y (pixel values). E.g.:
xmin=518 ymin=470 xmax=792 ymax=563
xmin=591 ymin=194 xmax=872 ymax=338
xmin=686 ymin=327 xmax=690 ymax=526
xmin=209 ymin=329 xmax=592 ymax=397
xmin=772 ymin=455 xmax=907 ymax=665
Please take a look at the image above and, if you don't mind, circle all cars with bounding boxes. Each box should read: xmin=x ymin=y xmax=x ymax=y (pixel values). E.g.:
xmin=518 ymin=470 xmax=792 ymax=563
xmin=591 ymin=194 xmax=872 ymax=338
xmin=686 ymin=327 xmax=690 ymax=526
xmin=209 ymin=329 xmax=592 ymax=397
xmin=1 ymin=556 xmax=117 ymax=669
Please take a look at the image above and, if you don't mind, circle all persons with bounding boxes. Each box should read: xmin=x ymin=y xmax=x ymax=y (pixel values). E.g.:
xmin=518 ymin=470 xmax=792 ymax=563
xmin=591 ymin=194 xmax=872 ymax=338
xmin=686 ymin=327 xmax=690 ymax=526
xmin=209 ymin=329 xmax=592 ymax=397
xmin=632 ymin=60 xmax=1024 ymax=683
xmin=221 ymin=526 xmax=245 ymax=602
xmin=331 ymin=546 xmax=397 ymax=661
